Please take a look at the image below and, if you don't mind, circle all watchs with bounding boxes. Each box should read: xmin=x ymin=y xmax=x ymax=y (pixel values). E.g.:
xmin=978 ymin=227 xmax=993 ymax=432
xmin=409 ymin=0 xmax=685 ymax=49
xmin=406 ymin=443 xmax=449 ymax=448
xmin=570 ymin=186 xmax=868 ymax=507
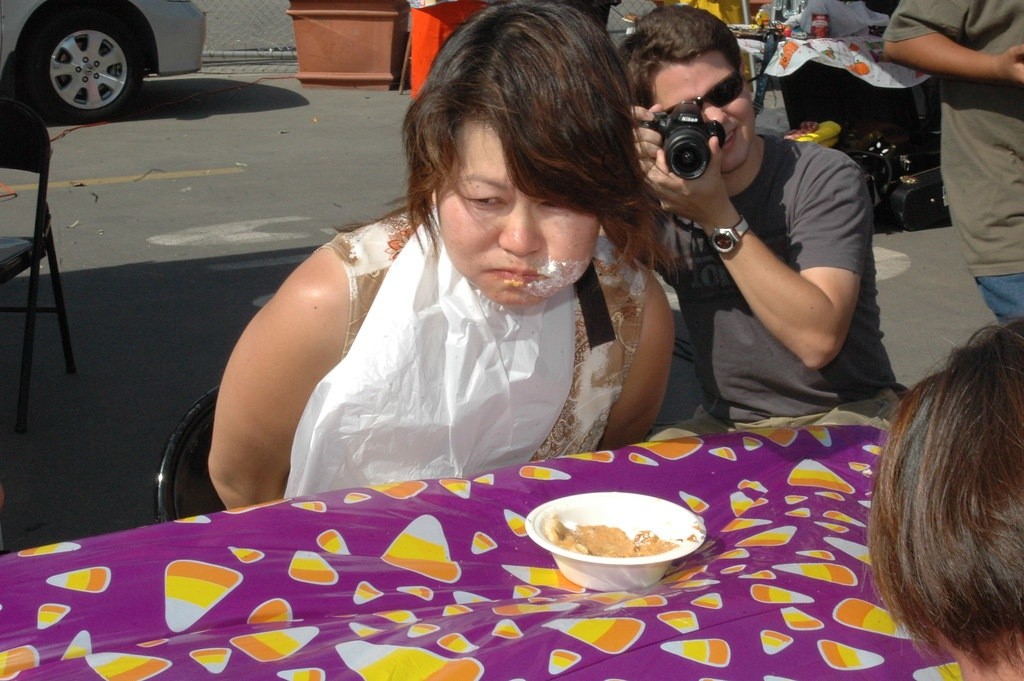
xmin=711 ymin=213 xmax=750 ymax=254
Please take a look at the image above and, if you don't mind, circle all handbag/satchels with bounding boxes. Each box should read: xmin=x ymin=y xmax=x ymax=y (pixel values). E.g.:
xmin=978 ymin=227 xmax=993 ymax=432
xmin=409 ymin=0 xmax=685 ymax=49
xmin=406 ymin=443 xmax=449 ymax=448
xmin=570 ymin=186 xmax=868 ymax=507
xmin=797 ymin=0 xmax=889 ymax=36
xmin=874 ymin=122 xmax=950 ymax=232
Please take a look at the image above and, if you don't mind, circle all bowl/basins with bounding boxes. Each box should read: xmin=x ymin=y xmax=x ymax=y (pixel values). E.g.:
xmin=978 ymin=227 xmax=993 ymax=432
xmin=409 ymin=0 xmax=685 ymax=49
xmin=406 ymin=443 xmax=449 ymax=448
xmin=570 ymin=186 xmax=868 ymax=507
xmin=525 ymin=491 xmax=707 ymax=592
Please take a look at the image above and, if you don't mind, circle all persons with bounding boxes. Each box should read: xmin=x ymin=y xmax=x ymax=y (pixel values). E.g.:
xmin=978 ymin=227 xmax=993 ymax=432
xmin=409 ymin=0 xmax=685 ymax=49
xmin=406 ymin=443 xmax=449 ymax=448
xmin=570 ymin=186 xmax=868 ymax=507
xmin=870 ymin=321 xmax=1024 ymax=680
xmin=207 ymin=0 xmax=675 ymax=515
xmin=617 ymin=0 xmax=1024 ymax=442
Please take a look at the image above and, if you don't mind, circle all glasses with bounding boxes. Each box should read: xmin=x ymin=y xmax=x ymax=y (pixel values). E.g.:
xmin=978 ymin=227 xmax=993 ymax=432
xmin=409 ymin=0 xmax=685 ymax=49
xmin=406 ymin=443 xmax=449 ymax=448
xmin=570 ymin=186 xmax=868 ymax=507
xmin=663 ymin=70 xmax=743 ymax=118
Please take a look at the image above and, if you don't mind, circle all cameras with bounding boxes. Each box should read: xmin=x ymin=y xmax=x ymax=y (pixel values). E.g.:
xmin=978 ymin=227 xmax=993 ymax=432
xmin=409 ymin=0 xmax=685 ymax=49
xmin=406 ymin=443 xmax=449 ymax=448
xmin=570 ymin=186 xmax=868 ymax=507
xmin=641 ymin=100 xmax=725 ymax=179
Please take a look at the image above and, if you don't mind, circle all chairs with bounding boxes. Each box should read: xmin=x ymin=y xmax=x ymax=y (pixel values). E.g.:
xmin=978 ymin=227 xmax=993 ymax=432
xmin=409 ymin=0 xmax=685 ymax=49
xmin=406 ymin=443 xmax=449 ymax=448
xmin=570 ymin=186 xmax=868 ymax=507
xmin=0 ymin=98 xmax=78 ymax=434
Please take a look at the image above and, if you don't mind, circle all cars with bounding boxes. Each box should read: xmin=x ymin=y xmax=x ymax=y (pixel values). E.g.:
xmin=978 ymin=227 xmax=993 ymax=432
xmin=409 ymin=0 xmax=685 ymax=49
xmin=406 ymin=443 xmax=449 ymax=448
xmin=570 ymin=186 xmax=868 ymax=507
xmin=1 ymin=0 xmax=205 ymax=126
